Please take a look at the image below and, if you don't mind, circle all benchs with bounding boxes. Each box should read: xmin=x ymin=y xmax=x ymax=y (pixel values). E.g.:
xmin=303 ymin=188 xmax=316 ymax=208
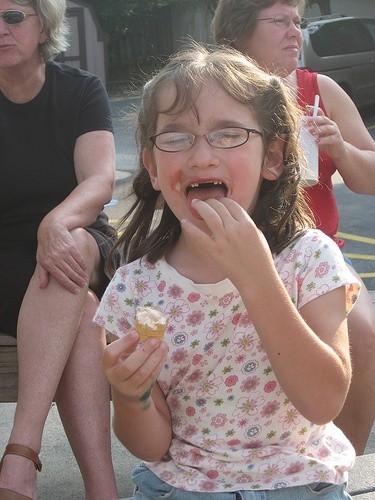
xmin=1 ymin=330 xmax=113 ymax=403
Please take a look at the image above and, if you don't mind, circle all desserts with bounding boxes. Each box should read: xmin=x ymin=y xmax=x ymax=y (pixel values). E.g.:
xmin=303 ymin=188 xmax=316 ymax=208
xmin=132 ymin=305 xmax=169 ymax=344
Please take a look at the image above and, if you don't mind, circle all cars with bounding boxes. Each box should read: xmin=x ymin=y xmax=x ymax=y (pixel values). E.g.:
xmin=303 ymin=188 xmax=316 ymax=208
xmin=296 ymin=13 xmax=375 ymax=112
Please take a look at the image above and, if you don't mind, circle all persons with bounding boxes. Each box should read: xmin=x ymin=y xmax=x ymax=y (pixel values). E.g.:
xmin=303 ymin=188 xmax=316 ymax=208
xmin=0 ymin=0 xmax=133 ymax=500
xmin=198 ymin=0 xmax=375 ymax=469
xmin=89 ymin=44 xmax=361 ymax=500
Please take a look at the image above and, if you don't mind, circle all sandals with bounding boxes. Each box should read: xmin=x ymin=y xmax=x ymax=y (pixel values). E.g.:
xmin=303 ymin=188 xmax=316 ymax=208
xmin=0 ymin=443 xmax=43 ymax=500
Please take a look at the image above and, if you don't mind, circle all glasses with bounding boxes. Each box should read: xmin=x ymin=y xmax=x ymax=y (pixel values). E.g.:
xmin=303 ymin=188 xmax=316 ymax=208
xmin=257 ymin=14 xmax=301 ymax=28
xmin=149 ymin=125 xmax=264 ymax=153
xmin=0 ymin=10 xmax=37 ymax=25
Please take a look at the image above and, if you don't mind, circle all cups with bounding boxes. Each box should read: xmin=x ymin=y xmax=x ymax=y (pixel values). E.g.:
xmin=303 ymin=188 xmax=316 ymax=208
xmin=295 ymin=116 xmax=318 ymax=186
